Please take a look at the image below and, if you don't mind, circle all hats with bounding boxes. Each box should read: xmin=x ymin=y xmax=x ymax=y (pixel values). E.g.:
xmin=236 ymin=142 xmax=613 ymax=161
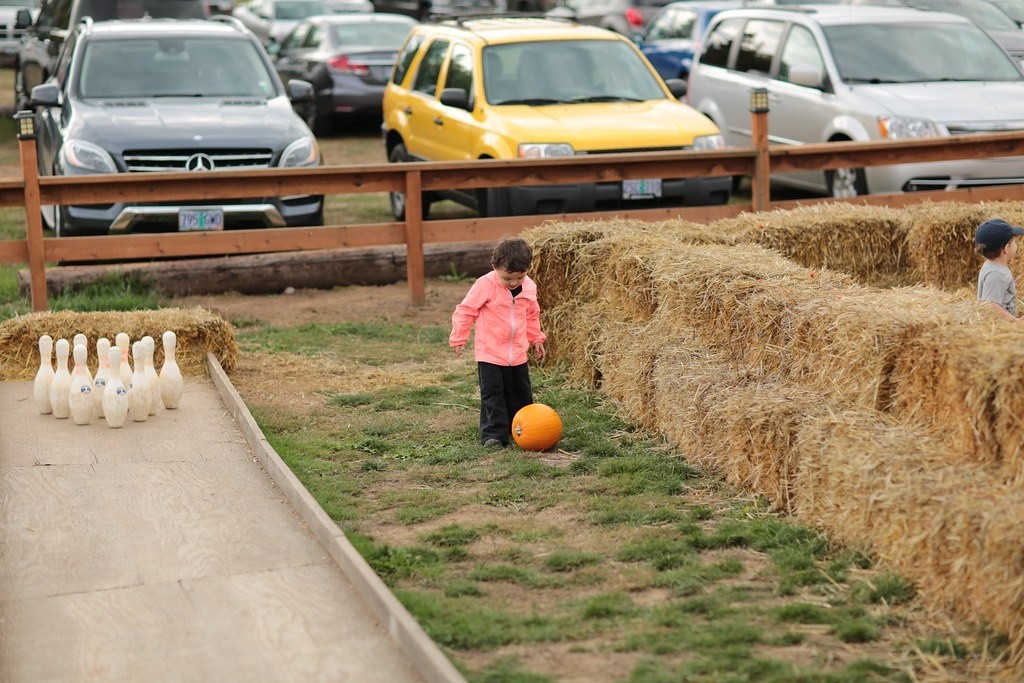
xmin=975 ymin=219 xmax=1024 ymax=243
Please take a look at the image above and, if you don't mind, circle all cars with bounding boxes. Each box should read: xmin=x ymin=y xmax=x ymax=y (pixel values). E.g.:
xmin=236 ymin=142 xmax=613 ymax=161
xmin=0 ymin=0 xmax=42 ymax=59
xmin=543 ymin=0 xmax=675 ymax=36
xmin=843 ymin=0 xmax=1023 ymax=78
xmin=233 ymin=0 xmax=376 ymax=63
xmin=265 ymin=12 xmax=424 ymax=133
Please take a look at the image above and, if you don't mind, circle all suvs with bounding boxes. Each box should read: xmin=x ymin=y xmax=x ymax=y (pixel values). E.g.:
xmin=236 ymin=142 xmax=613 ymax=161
xmin=13 ymin=1 xmax=208 ymax=117
xmin=382 ymin=11 xmax=735 ymax=216
xmin=31 ymin=13 xmax=324 ymax=266
xmin=622 ymin=0 xmax=775 ymax=99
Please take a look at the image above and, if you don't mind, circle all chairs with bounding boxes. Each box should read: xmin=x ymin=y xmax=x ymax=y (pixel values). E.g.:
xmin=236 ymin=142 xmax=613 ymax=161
xmin=86 ymin=52 xmax=252 ymax=97
xmin=467 ymin=48 xmax=617 ymax=104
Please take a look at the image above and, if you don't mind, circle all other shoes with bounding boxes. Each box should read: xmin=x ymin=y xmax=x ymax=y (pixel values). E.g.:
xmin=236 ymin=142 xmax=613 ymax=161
xmin=484 ymin=439 xmax=502 ymax=448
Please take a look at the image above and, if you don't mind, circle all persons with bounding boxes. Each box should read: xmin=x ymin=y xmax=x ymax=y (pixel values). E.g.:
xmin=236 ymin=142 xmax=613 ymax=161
xmin=974 ymin=219 xmax=1024 ymax=315
xmin=448 ymin=239 xmax=548 ymax=448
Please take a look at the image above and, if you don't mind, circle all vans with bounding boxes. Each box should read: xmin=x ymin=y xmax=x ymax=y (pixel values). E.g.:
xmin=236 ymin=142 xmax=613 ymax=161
xmin=683 ymin=4 xmax=1023 ymax=200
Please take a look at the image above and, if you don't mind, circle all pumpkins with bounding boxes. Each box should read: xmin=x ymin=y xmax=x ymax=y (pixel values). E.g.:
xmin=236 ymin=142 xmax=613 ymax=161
xmin=512 ymin=403 xmax=562 ymax=451
